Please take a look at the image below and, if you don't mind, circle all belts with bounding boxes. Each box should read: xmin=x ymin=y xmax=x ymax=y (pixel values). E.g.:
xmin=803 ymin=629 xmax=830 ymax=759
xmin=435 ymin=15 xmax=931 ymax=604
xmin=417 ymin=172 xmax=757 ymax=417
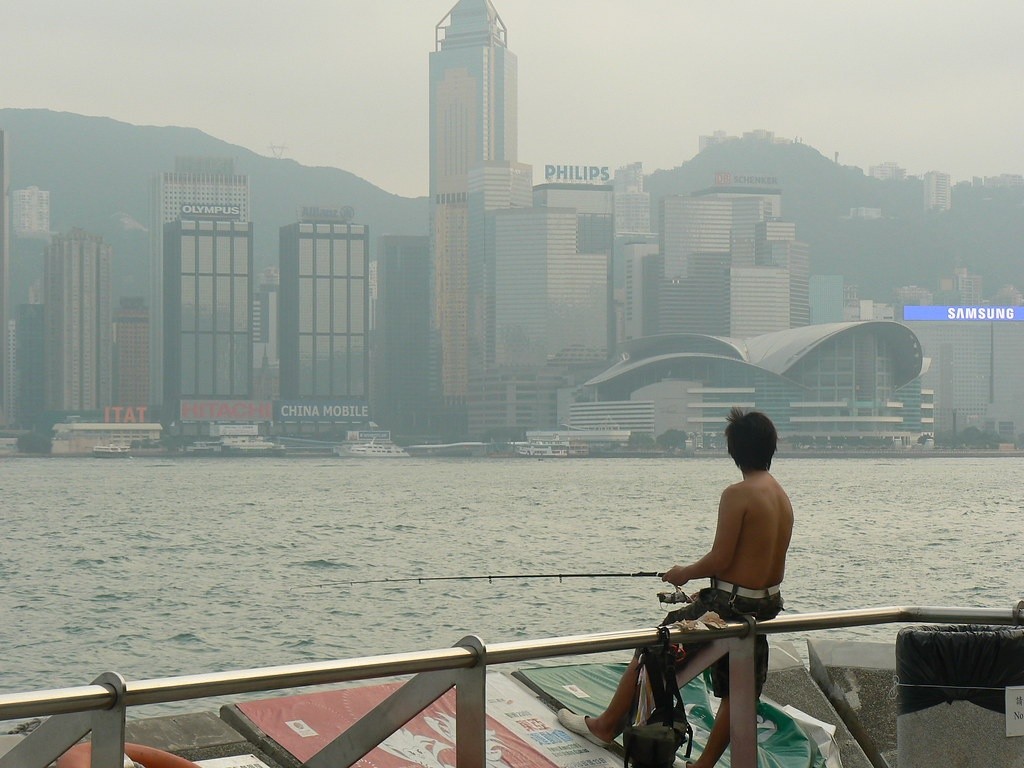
xmin=710 ymin=579 xmax=781 ymax=599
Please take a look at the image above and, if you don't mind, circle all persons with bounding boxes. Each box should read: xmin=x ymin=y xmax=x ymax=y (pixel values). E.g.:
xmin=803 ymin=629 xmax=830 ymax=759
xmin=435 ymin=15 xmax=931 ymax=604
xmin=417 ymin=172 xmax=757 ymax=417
xmin=556 ymin=405 xmax=794 ymax=768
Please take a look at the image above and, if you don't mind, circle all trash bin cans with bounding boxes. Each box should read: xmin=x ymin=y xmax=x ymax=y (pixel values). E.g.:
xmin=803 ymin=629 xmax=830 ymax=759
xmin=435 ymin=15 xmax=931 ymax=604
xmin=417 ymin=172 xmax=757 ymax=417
xmin=895 ymin=623 xmax=1024 ymax=768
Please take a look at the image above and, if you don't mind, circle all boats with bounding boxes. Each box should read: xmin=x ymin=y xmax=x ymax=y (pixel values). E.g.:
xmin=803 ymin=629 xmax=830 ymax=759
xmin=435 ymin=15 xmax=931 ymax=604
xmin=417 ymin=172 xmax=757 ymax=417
xmin=333 ymin=430 xmax=409 ymax=458
xmin=93 ymin=443 xmax=130 ymax=459
xmin=518 ymin=433 xmax=569 ymax=458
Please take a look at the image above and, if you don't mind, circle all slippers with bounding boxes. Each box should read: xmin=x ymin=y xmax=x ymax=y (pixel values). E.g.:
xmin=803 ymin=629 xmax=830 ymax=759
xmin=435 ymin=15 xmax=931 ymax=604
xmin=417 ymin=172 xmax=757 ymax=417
xmin=673 ymin=756 xmax=692 ymax=768
xmin=557 ymin=708 xmax=611 ymax=748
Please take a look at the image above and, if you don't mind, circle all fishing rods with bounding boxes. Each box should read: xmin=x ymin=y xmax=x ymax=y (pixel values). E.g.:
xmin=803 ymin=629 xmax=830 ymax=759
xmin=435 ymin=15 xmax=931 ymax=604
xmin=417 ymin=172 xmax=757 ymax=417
xmin=287 ymin=572 xmax=669 ymax=588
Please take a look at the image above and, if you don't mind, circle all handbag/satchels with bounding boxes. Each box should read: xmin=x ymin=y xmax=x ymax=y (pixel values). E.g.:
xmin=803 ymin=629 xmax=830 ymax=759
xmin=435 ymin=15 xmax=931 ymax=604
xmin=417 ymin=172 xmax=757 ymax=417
xmin=620 ymin=705 xmax=694 ymax=768
